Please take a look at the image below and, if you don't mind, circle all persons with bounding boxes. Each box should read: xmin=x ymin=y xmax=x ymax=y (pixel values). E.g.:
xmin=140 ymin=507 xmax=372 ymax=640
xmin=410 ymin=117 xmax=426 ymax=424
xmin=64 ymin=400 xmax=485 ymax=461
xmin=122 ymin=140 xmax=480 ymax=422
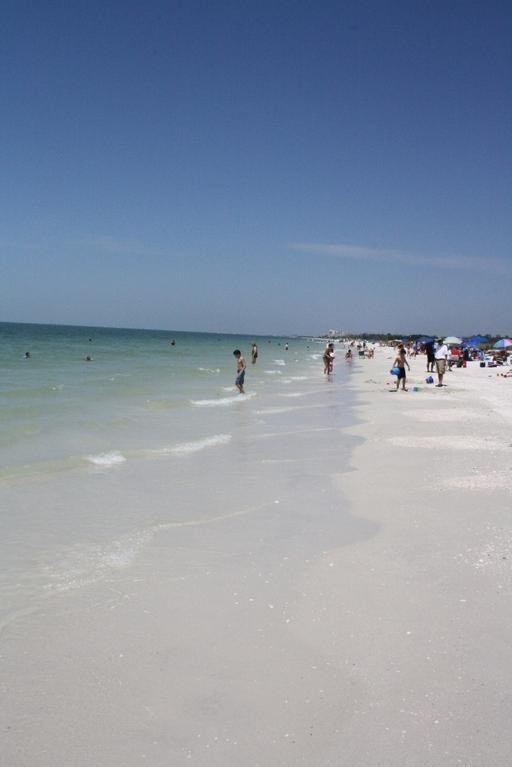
xmin=316 ymin=335 xmax=512 ymax=375
xmin=285 ymin=341 xmax=289 ymax=351
xmin=391 ymin=347 xmax=411 ymax=392
xmin=433 ymin=336 xmax=448 ymax=387
xmin=231 ymin=349 xmax=247 ymax=394
xmin=249 ymin=341 xmax=258 ymax=364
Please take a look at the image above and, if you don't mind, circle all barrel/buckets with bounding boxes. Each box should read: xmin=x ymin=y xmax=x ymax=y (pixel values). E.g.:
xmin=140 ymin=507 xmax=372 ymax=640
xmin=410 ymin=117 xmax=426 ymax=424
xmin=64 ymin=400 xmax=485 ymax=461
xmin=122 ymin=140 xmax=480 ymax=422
xmin=390 ymin=368 xmax=400 ymax=375
xmin=426 ymin=376 xmax=433 ymax=383
